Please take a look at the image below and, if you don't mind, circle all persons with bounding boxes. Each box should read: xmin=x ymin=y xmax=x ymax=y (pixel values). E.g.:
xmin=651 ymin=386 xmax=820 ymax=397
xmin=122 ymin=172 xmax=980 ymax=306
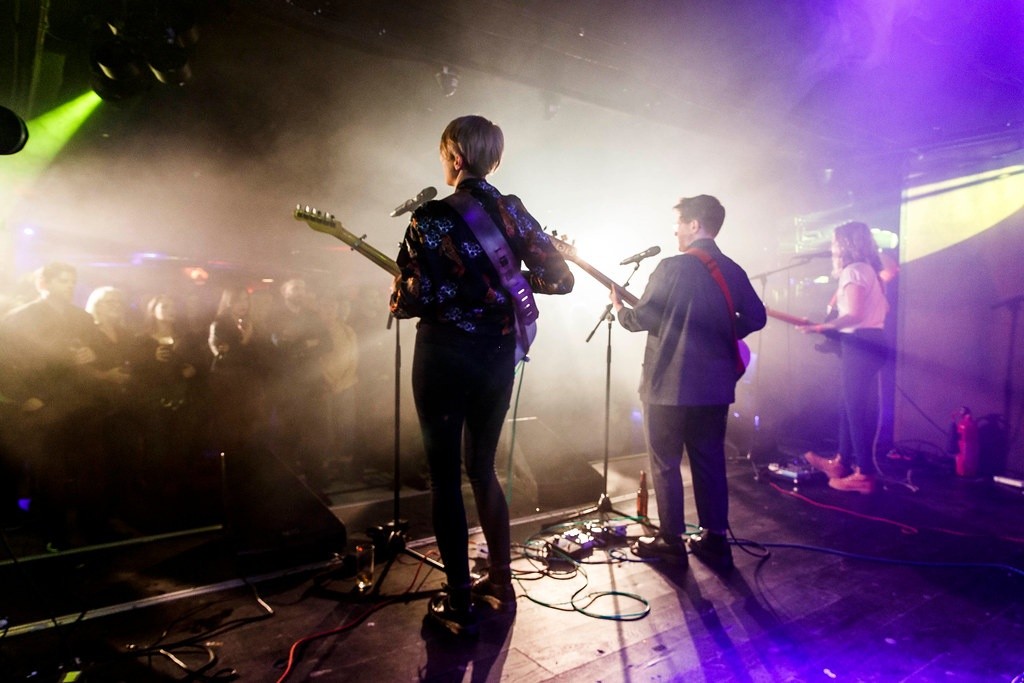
xmin=802 ymin=219 xmax=891 ymax=496
xmin=611 ymin=193 xmax=767 ymax=565
xmin=3 ymin=275 xmax=364 ymax=470
xmin=387 ymin=114 xmax=575 ymax=631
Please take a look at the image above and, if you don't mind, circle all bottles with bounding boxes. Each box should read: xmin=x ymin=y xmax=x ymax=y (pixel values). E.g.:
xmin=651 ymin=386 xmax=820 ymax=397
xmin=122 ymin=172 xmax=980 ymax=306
xmin=637 ymin=470 xmax=649 ymax=516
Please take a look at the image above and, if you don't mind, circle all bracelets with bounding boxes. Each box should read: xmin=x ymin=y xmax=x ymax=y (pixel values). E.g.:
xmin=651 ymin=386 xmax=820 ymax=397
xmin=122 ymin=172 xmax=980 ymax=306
xmin=814 ymin=324 xmax=822 ymax=332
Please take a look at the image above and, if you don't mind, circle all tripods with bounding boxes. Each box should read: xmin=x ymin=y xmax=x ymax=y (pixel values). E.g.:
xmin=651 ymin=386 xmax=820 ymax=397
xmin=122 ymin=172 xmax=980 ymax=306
xmin=726 ymin=257 xmax=810 ymax=483
xmin=540 ymin=262 xmax=660 ymax=530
xmin=353 ymin=319 xmax=482 ymax=609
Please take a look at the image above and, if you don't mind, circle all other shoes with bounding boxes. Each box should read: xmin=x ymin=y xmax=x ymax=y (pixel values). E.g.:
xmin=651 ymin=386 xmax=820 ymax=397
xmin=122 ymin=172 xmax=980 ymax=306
xmin=828 ymin=467 xmax=875 ymax=495
xmin=804 ymin=450 xmax=848 ymax=480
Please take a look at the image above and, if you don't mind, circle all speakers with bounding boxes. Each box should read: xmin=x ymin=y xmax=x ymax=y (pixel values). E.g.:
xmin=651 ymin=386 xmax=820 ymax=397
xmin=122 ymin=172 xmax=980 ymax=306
xmin=498 ymin=417 xmax=603 ymax=510
xmin=219 ymin=445 xmax=348 ymax=558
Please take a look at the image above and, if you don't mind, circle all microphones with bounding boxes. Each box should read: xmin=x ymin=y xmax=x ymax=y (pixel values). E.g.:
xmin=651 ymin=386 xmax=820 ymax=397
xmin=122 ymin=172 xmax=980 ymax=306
xmin=794 ymin=250 xmax=833 ymax=258
xmin=621 ymin=246 xmax=661 ymax=265
xmin=389 ymin=186 xmax=437 ymax=217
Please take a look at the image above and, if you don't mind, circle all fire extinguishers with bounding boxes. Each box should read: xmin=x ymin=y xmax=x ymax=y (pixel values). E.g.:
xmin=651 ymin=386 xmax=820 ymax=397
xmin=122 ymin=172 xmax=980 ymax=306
xmin=946 ymin=405 xmax=980 ymax=477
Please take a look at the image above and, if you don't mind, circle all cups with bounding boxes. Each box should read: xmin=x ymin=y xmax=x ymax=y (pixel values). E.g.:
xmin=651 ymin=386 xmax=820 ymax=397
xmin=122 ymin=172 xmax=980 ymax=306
xmin=356 ymin=544 xmax=375 ymax=587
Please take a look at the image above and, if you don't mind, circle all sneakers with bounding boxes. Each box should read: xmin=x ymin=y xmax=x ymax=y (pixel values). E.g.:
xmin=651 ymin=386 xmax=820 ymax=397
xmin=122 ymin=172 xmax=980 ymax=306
xmin=634 ymin=536 xmax=688 ymax=560
xmin=471 ymin=573 xmax=517 ymax=612
xmin=687 ymin=533 xmax=732 ymax=564
xmin=428 ymin=591 xmax=481 ymax=637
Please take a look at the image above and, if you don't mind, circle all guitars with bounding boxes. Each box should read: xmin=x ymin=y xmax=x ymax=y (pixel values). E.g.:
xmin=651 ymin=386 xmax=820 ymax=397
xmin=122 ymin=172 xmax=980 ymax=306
xmin=292 ymin=202 xmax=540 ymax=368
xmin=537 ymin=223 xmax=753 ymax=384
xmin=765 ymin=303 xmax=844 ymax=358
